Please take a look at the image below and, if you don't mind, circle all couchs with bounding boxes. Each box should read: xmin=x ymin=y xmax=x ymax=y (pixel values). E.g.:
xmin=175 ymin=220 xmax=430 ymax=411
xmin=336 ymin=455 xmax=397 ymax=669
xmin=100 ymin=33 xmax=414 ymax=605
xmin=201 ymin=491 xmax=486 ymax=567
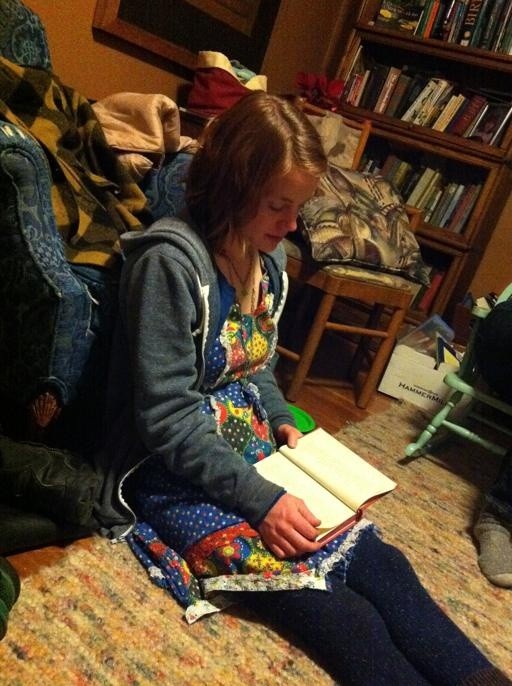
xmin=0 ymin=0 xmax=287 ymax=439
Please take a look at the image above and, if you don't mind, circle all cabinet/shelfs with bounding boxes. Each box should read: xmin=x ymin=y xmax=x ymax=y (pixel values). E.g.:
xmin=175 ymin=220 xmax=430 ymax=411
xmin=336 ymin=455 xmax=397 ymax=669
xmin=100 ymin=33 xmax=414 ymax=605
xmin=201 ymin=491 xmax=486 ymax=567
xmin=278 ymin=1 xmax=511 ymax=366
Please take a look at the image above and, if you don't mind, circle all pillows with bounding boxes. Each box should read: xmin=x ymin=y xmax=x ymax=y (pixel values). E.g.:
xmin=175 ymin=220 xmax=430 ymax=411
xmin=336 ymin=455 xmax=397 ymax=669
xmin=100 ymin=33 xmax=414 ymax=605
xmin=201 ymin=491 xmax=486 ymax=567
xmin=299 ymin=166 xmax=430 ymax=289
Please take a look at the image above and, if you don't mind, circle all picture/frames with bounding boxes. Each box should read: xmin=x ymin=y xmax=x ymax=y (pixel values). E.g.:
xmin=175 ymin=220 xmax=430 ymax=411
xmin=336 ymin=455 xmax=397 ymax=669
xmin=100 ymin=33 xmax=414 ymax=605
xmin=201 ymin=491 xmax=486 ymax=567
xmin=90 ymin=1 xmax=280 ymax=92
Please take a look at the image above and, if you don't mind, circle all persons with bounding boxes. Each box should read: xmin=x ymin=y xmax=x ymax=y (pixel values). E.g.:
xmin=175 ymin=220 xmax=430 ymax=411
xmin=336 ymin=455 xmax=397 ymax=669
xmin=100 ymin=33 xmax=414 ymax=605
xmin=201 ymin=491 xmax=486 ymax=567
xmin=472 ymin=300 xmax=512 ymax=588
xmin=94 ymin=90 xmax=510 ymax=686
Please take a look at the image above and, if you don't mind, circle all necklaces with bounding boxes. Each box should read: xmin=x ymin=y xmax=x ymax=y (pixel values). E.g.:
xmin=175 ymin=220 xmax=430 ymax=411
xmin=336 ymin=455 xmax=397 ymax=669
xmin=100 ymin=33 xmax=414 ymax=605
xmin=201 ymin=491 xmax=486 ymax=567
xmin=219 ymin=250 xmax=255 ymax=296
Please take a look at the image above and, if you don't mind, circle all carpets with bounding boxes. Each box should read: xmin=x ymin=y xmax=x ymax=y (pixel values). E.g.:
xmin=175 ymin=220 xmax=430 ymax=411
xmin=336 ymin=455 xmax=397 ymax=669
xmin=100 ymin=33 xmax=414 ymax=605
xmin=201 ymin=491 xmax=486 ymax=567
xmin=0 ymin=401 xmax=511 ymax=686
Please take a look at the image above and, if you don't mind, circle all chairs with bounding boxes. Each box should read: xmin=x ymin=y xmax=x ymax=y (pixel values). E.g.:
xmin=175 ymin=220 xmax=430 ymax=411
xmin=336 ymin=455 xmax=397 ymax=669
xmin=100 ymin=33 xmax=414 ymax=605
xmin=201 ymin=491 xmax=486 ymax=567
xmin=403 ymin=281 xmax=511 ymax=460
xmin=276 ymin=95 xmax=424 ymax=410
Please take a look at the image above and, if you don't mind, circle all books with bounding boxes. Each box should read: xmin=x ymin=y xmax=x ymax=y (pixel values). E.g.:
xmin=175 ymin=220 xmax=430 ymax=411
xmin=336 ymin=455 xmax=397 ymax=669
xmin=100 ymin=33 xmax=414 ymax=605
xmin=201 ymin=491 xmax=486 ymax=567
xmin=357 ymin=153 xmax=483 ymax=237
xmin=409 ymin=262 xmax=445 ymax=314
xmin=376 ymin=0 xmax=512 ymax=56
xmin=341 ymin=44 xmax=511 ymax=151
xmin=252 ymin=427 xmax=399 ymax=547
